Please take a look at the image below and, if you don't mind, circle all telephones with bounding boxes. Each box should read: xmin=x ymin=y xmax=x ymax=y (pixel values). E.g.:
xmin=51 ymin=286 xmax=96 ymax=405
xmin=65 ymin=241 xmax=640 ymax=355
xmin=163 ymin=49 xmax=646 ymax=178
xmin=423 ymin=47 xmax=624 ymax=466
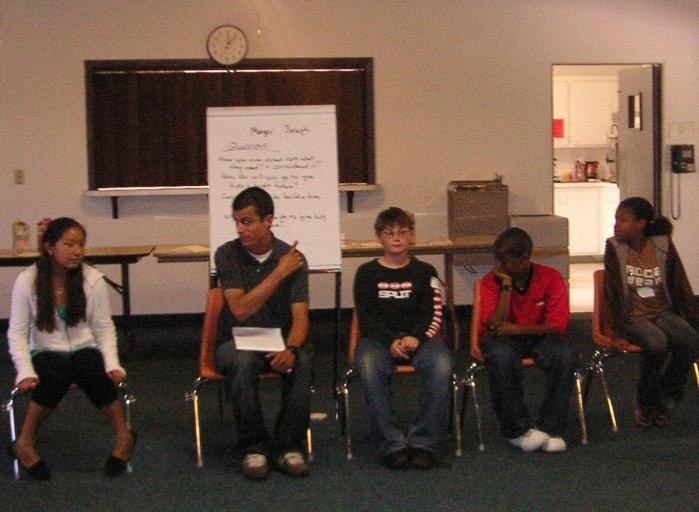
xmin=670 ymin=144 xmax=696 ymax=173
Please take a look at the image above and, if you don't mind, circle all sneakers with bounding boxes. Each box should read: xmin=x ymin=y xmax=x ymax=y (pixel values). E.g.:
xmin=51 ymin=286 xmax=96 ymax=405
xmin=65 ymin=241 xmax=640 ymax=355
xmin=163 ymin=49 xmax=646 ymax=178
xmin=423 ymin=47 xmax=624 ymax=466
xmin=508 ymin=428 xmax=548 ymax=451
xmin=243 ymin=453 xmax=270 ymax=480
xmin=542 ymin=434 xmax=566 ymax=452
xmin=408 ymin=448 xmax=433 ymax=470
xmin=385 ymin=448 xmax=411 ymax=468
xmin=278 ymin=449 xmax=309 ymax=478
xmin=633 ymin=393 xmax=653 ymax=428
xmin=651 ymin=404 xmax=669 ymax=427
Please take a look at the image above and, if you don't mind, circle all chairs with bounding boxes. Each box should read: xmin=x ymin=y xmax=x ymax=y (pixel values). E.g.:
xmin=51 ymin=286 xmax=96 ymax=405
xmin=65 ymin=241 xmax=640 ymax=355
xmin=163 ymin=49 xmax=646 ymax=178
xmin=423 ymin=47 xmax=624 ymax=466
xmin=587 ymin=267 xmax=697 ymax=439
xmin=339 ymin=296 xmax=464 ymax=461
xmin=175 ymin=288 xmax=356 ymax=467
xmin=468 ymin=274 xmax=591 ymax=453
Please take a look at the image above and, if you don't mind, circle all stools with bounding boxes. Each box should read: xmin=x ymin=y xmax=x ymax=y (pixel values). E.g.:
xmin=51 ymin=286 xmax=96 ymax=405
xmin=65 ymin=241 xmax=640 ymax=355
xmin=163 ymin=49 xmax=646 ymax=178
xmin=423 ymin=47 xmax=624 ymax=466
xmin=4 ymin=375 xmax=145 ymax=476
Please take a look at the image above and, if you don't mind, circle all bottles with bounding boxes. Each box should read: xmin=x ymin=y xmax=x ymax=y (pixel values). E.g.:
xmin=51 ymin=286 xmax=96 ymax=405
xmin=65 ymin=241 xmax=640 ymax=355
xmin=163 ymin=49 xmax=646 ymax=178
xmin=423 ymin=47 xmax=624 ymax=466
xmin=36 ymin=216 xmax=54 ymax=252
xmin=12 ymin=218 xmax=30 ymax=256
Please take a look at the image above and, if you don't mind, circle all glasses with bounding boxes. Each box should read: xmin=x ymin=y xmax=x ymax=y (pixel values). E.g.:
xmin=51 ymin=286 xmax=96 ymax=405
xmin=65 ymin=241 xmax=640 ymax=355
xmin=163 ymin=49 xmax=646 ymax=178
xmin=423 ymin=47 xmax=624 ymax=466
xmin=382 ymin=230 xmax=410 ymax=237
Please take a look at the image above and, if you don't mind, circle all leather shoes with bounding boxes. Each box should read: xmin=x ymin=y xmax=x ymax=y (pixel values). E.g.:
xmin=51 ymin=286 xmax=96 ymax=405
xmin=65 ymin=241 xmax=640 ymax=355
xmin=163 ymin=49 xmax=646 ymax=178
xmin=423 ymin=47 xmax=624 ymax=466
xmin=9 ymin=438 xmax=52 ymax=480
xmin=107 ymin=429 xmax=138 ymax=471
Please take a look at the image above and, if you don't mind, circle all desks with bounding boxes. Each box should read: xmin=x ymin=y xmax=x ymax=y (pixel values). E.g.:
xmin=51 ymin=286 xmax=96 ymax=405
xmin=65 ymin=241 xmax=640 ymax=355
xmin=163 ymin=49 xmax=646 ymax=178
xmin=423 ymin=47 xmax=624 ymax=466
xmin=344 ymin=234 xmax=566 ymax=352
xmin=160 ymin=238 xmax=209 ymax=264
xmin=3 ymin=235 xmax=160 ymax=360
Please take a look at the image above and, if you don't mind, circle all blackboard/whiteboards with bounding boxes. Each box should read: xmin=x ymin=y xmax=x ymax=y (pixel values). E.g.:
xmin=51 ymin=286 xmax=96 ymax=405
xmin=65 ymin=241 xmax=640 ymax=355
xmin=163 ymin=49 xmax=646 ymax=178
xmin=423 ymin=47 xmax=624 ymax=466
xmin=206 ymin=104 xmax=342 ymax=275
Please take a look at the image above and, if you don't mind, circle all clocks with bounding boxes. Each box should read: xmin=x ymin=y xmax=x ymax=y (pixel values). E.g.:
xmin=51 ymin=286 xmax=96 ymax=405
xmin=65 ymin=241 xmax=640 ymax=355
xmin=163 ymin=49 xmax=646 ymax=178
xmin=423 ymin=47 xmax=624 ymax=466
xmin=202 ymin=25 xmax=250 ymax=68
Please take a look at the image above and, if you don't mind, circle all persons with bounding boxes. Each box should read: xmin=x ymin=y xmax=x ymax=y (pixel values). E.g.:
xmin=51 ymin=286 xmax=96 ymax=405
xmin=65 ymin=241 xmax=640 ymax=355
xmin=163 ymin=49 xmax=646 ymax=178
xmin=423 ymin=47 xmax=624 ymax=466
xmin=213 ymin=186 xmax=316 ymax=480
xmin=474 ymin=226 xmax=574 ymax=455
xmin=598 ymin=197 xmax=699 ymax=429
xmin=4 ymin=215 xmax=139 ymax=479
xmin=352 ymin=207 xmax=453 ymax=468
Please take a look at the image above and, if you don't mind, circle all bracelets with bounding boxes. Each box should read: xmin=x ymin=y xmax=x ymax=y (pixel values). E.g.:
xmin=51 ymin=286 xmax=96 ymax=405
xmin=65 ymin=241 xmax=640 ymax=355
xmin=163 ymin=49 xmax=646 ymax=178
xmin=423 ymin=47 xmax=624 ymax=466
xmin=500 ymin=285 xmax=513 ymax=292
xmin=284 ymin=344 xmax=300 ymax=358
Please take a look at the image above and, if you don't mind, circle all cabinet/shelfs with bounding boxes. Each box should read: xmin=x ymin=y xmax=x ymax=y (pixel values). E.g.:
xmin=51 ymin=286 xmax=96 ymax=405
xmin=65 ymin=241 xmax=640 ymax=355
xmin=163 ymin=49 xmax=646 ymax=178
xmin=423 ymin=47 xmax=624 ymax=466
xmin=554 ymin=187 xmax=600 ymax=258
xmin=553 ymin=80 xmax=616 ymax=144
xmin=600 ymin=187 xmax=622 ymax=256
